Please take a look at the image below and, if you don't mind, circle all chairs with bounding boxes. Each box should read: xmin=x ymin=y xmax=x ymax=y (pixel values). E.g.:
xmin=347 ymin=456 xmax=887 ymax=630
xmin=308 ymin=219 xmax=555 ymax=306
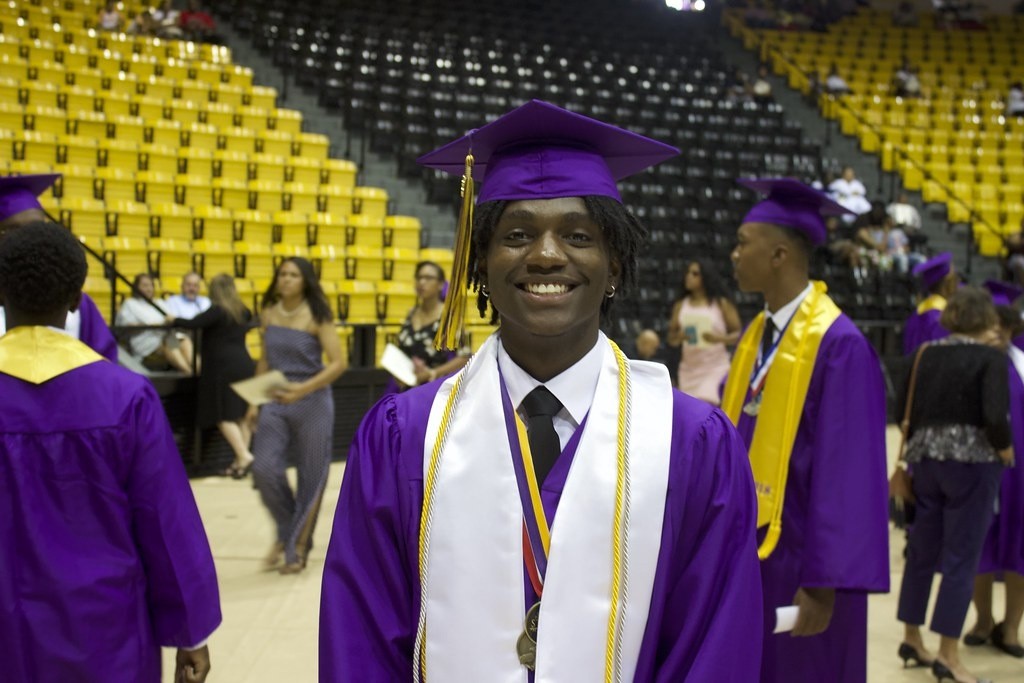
xmin=0 ymin=0 xmax=1024 ymax=367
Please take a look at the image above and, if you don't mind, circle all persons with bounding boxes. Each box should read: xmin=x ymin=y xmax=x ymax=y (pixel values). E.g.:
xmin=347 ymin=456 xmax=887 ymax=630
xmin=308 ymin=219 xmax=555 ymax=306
xmin=895 ymin=286 xmax=1015 ymax=683
xmin=717 ymin=177 xmax=891 ymax=683
xmin=165 ymin=273 xmax=212 ymax=320
xmin=245 ymin=255 xmax=348 ymax=575
xmin=115 ymin=273 xmax=193 ymax=375
xmin=903 ymin=252 xmax=955 ymax=355
xmin=0 ymin=173 xmax=118 ymax=365
xmin=666 ymin=255 xmax=743 ymax=408
xmin=964 ymin=278 xmax=1024 ymax=658
xmin=0 ymin=219 xmax=222 ymax=683
xmin=318 ymin=99 xmax=765 ymax=683
xmin=809 ymin=164 xmax=928 ymax=287
xmin=635 ymin=329 xmax=680 ymax=387
xmin=395 ymin=261 xmax=472 ymax=393
xmin=721 ymin=0 xmax=1024 ymax=119
xmin=163 ymin=273 xmax=254 ymax=479
xmin=98 ymin=0 xmax=227 ymax=46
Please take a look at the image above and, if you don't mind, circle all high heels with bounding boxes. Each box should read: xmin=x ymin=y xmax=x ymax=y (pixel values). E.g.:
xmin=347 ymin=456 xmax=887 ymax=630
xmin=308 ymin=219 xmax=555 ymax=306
xmin=899 ymin=642 xmax=944 ymax=668
xmin=932 ymin=659 xmax=959 ymax=682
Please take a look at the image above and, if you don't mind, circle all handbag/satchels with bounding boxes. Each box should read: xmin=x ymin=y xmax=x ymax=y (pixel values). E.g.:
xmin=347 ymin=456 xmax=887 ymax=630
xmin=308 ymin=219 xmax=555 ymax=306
xmin=888 ymin=465 xmax=916 ymax=528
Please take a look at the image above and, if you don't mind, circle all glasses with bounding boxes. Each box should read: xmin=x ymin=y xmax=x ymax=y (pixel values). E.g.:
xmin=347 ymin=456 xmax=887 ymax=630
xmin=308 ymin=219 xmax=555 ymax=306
xmin=417 ymin=274 xmax=440 ymax=280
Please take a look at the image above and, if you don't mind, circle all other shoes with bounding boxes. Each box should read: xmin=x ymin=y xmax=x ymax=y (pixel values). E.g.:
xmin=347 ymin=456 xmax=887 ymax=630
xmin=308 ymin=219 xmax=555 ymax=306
xmin=964 ymin=632 xmax=993 ymax=646
xmin=219 ymin=457 xmax=260 ymax=480
xmin=261 ymin=548 xmax=285 ymax=572
xmin=992 ymin=622 xmax=1024 ymax=658
xmin=280 ymin=544 xmax=313 ymax=575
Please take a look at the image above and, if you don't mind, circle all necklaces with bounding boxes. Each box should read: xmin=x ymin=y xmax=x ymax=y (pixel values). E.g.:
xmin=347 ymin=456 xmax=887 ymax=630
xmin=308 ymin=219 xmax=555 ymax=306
xmin=278 ymin=300 xmax=306 ymax=317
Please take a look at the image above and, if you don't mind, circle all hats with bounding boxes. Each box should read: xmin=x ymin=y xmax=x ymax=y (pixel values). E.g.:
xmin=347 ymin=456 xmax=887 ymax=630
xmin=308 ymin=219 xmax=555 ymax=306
xmin=911 ymin=253 xmax=953 ymax=286
xmin=415 ymin=98 xmax=681 ymax=351
xmin=742 ymin=177 xmax=862 ymax=252
xmin=0 ymin=169 xmax=61 ymax=222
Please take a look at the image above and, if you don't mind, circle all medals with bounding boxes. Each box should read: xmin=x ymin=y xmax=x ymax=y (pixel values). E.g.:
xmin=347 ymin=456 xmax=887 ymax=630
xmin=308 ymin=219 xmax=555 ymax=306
xmin=743 ymin=401 xmax=766 ymax=416
xmin=516 ymin=601 xmax=540 ymax=671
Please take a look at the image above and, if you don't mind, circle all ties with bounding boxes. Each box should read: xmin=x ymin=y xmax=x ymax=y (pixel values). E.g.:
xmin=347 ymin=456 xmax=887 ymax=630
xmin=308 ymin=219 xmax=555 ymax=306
xmin=763 ymin=315 xmax=775 ymax=350
xmin=516 ymin=383 xmax=564 ymax=487
